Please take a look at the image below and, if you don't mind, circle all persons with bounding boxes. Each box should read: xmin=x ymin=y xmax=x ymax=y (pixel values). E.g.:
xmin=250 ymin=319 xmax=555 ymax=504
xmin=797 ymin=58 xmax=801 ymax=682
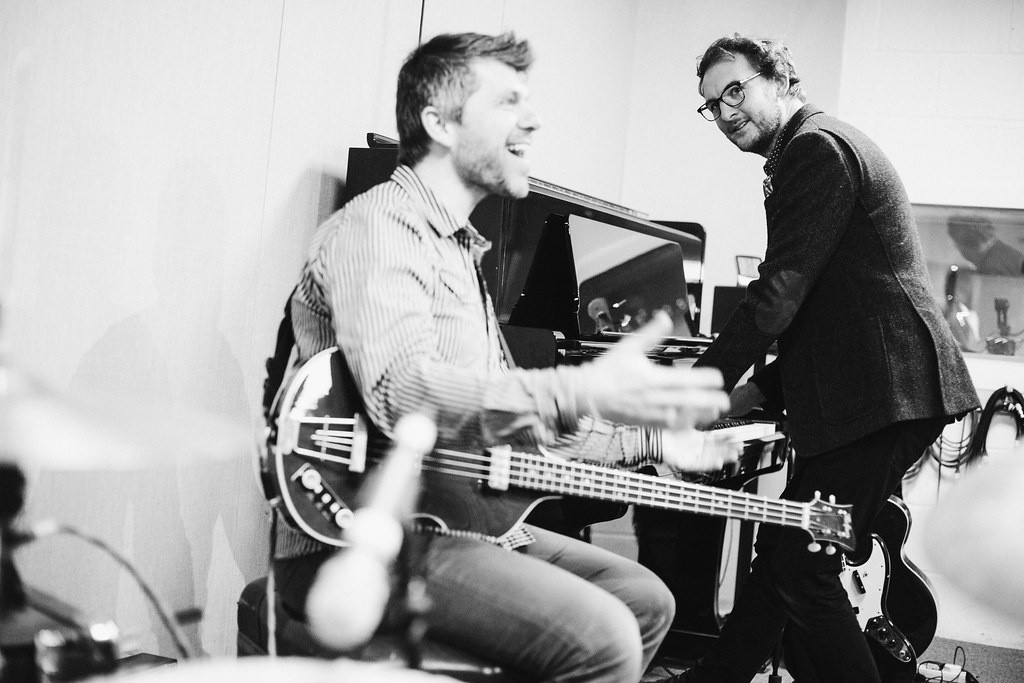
xmin=259 ymin=28 xmax=744 ymax=683
xmin=694 ymin=35 xmax=983 ymax=682
xmin=945 ymin=215 xmax=1024 ymax=276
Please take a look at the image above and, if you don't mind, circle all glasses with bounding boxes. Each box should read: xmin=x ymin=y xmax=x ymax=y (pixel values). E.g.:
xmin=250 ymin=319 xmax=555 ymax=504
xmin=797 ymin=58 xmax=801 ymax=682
xmin=697 ymin=71 xmax=764 ymax=121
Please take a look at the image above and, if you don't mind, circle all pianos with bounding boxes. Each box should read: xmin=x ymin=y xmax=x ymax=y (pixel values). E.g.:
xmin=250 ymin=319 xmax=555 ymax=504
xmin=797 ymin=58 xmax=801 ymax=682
xmin=331 ymin=132 xmax=792 ymax=668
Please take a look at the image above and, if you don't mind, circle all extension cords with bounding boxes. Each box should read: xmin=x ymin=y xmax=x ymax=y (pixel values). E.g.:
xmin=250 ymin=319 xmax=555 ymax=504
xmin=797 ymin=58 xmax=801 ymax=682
xmin=915 ymin=663 xmax=967 ymax=683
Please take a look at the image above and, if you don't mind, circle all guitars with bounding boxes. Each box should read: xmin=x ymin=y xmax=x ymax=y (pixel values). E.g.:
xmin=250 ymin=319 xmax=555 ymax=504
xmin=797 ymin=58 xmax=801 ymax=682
xmin=262 ymin=344 xmax=862 ymax=561
